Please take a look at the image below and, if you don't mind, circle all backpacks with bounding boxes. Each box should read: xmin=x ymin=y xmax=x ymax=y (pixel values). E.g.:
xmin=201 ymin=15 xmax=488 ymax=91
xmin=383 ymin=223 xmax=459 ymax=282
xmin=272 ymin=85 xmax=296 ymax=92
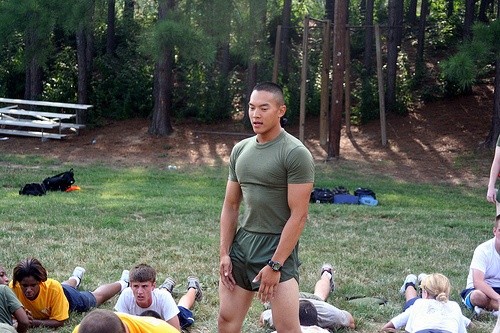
xmin=313 ymin=188 xmax=333 ymax=203
xmin=19 ymin=183 xmax=46 ymax=196
xmin=360 ymin=196 xmax=378 ymax=206
xmin=42 ymin=168 xmax=75 ymax=191
xmin=334 ymin=186 xmax=349 ymax=194
xmin=334 ymin=194 xmax=358 ymax=204
xmin=355 ymin=188 xmax=375 ymax=205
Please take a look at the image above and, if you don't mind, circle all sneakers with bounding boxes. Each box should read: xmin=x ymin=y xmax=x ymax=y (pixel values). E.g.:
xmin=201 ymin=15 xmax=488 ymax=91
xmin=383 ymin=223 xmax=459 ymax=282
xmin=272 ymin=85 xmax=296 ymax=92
xmin=188 ymin=276 xmax=202 ymax=302
xmin=159 ymin=278 xmax=176 ymax=292
xmin=418 ymin=273 xmax=427 ymax=298
xmin=321 ymin=265 xmax=334 ymax=292
xmin=121 ymin=270 xmax=130 ymax=287
xmin=72 ymin=267 xmax=85 ymax=288
xmin=400 ymin=275 xmax=416 ymax=296
